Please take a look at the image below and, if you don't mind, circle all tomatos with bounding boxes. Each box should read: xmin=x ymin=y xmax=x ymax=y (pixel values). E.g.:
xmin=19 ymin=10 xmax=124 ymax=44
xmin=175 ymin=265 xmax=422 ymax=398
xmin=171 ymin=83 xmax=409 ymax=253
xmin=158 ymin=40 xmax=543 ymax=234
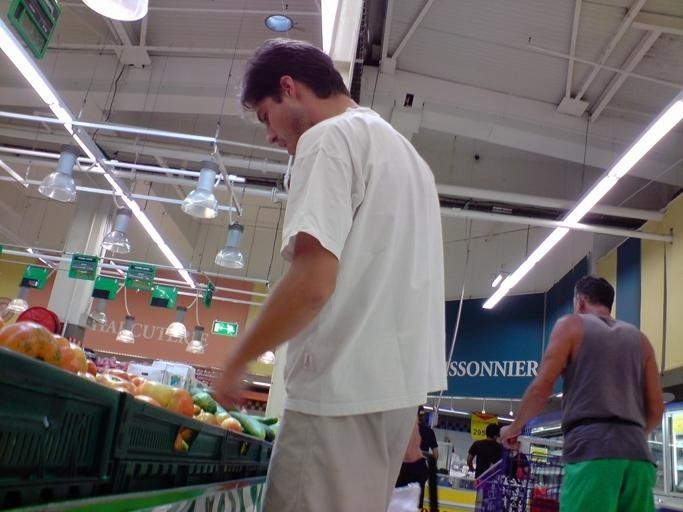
xmin=0 ymin=321 xmax=244 ymax=453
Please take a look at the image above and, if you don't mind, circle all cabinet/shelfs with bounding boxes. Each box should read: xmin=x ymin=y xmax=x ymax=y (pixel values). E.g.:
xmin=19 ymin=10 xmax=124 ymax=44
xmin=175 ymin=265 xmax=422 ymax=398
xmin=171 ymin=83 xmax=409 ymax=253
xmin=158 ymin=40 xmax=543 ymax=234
xmin=0 ymin=364 xmax=274 ymax=512
xmin=420 ymin=403 xmax=683 ymax=511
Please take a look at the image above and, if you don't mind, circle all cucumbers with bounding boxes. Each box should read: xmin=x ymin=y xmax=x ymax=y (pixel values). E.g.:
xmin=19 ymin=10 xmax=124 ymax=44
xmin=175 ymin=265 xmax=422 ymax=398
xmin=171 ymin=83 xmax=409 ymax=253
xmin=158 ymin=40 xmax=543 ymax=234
xmin=192 ymin=392 xmax=278 ymax=442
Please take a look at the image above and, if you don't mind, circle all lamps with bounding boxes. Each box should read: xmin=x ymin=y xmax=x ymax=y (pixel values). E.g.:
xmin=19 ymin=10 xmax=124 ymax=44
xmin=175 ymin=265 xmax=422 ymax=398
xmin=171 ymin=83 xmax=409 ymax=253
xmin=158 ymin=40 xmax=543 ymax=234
xmin=82 ymin=0 xmax=148 ymax=22
xmin=0 ymin=18 xmax=197 ymax=290
xmin=185 ymin=326 xmax=205 ymax=353
xmin=90 ymin=297 xmax=107 ymax=324
xmin=5 ymin=286 xmax=29 ymax=313
xmin=481 ymin=88 xmax=682 ymax=310
xmin=166 ymin=307 xmax=187 ymax=337
xmin=181 ymin=145 xmax=246 ymax=269
xmin=99 ymin=208 xmax=132 ymax=254
xmin=491 ymin=270 xmax=505 ymax=289
xmin=38 ymin=146 xmax=77 ymax=205
xmin=116 ymin=316 xmax=136 ymax=345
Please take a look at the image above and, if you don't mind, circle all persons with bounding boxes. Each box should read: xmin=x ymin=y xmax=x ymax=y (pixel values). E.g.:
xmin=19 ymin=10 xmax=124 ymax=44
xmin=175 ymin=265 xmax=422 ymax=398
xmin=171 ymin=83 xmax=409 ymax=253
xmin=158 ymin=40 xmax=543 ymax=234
xmin=213 ymin=38 xmax=448 ymax=512
xmin=499 ymin=275 xmax=665 ymax=512
xmin=467 ymin=423 xmax=503 ymax=478
xmin=396 ymin=411 xmax=438 ymax=512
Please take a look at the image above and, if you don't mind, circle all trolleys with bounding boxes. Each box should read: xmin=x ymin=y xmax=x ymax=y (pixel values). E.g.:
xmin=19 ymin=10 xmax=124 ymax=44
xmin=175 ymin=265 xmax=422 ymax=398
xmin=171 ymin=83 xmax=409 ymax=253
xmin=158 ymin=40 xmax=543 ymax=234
xmin=469 ymin=429 xmax=563 ymax=512
